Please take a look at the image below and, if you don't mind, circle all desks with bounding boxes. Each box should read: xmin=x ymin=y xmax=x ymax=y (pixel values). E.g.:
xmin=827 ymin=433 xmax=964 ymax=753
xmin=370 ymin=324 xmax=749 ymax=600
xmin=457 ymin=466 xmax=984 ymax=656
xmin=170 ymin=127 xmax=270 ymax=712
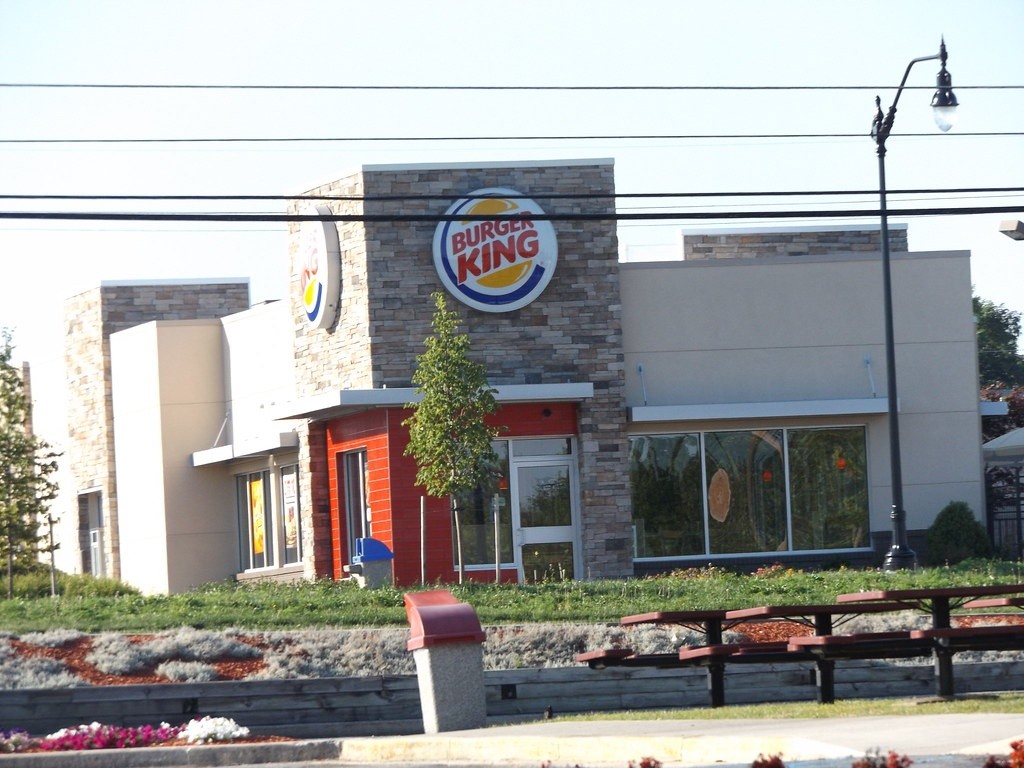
xmin=621 ymin=608 xmax=739 ymax=707
xmin=836 ymin=584 xmax=1024 ymax=698
xmin=726 ymin=601 xmax=922 ymax=704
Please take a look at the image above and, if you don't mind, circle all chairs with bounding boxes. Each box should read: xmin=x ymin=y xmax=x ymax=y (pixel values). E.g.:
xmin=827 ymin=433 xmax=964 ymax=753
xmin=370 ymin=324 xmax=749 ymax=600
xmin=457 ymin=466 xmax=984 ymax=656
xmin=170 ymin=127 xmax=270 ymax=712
xmin=964 ymin=597 xmax=1024 ymax=610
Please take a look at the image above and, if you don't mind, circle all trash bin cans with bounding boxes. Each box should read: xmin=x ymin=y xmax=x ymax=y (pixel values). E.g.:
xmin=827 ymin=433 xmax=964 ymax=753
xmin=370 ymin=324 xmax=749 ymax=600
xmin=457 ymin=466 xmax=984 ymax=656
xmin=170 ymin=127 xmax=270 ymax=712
xmin=342 ymin=538 xmax=395 ymax=590
xmin=402 ymin=590 xmax=486 ymax=733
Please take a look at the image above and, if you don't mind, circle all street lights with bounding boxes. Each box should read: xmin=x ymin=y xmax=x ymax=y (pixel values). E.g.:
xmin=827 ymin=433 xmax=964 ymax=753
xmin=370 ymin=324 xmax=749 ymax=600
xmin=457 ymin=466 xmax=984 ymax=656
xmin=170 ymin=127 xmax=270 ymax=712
xmin=869 ymin=37 xmax=961 ymax=573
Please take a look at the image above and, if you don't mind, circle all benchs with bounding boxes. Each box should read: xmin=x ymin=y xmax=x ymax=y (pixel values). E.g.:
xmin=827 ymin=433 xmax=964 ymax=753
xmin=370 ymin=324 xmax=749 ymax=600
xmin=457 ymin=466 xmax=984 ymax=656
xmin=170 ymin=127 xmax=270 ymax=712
xmin=575 ymin=625 xmax=1024 ymax=670
